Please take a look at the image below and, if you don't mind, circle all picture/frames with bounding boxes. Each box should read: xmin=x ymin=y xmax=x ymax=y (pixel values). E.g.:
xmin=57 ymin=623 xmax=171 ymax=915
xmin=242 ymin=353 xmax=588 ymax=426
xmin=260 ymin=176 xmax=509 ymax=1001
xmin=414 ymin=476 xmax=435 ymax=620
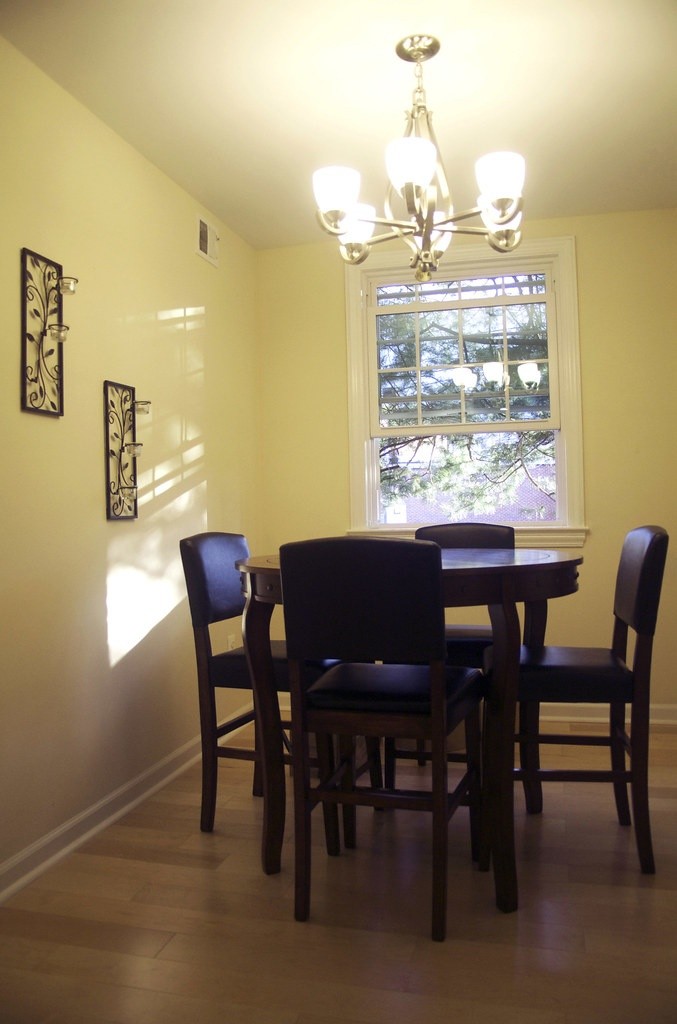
xmin=104 ymin=378 xmax=139 ymax=522
xmin=18 ymin=246 xmax=64 ymax=417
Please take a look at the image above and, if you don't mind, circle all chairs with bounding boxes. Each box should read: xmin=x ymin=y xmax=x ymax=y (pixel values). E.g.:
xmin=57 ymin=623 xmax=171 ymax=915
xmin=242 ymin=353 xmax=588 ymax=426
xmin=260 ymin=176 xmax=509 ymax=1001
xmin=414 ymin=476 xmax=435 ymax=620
xmin=485 ymin=525 xmax=669 ymax=877
xmin=273 ymin=532 xmax=491 ymax=946
xmin=399 ymin=517 xmax=521 ymax=778
xmin=178 ymin=533 xmax=384 ymax=855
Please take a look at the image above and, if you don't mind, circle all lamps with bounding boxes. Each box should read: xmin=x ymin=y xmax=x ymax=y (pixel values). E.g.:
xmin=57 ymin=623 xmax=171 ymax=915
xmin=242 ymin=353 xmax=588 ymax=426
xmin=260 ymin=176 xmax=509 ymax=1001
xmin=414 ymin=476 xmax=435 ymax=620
xmin=311 ymin=32 xmax=528 ymax=281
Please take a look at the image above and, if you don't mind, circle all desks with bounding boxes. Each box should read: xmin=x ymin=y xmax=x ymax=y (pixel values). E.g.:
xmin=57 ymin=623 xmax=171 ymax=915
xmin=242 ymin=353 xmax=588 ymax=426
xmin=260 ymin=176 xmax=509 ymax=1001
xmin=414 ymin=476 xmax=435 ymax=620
xmin=233 ymin=542 xmax=587 ymax=919
